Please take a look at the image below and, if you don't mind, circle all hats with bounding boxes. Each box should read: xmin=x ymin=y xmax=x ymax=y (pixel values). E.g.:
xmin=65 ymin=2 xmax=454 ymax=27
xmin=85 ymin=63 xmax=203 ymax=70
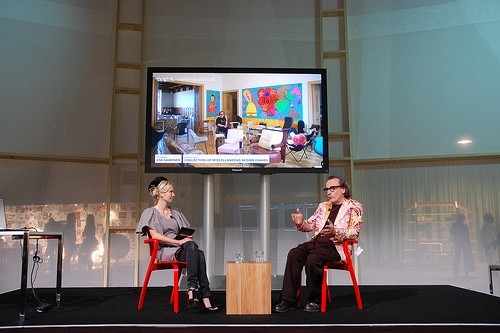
xmin=148 ymin=176 xmax=168 ymax=196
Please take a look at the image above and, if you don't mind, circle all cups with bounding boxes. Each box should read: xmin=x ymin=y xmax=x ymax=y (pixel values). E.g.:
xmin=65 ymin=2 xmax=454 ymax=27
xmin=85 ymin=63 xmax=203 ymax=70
xmin=233 ymin=251 xmax=243 ymax=263
xmin=255 ymin=250 xmax=263 ymax=262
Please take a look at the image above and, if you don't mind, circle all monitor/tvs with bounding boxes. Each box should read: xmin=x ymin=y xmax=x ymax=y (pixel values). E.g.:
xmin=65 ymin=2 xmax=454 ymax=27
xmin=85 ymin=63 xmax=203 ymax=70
xmin=144 ymin=67 xmax=330 ymax=174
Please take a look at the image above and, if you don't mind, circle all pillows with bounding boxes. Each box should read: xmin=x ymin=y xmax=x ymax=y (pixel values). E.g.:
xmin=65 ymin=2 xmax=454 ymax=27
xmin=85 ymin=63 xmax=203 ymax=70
xmin=290 ymin=132 xmax=306 ymax=147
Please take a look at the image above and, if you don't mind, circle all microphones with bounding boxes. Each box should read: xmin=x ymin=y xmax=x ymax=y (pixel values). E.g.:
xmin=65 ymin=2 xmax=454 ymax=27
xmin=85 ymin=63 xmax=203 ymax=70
xmin=170 ymin=216 xmax=173 ymax=218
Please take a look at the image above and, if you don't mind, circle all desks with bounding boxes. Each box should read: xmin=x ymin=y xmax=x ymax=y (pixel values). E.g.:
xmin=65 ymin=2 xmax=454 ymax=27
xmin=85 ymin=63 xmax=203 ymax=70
xmin=226 ymin=260 xmax=272 ymax=315
xmin=0 ymin=228 xmax=63 ymax=325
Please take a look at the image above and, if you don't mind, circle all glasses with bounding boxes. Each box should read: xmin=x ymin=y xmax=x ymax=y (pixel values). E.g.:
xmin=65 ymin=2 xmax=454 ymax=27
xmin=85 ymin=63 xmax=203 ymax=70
xmin=323 ymin=185 xmax=341 ymax=192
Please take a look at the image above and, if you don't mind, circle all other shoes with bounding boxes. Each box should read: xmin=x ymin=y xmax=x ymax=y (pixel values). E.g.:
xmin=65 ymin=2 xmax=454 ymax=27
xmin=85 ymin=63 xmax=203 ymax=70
xmin=272 ymin=301 xmax=296 ymax=313
xmin=304 ymin=302 xmax=319 ymax=312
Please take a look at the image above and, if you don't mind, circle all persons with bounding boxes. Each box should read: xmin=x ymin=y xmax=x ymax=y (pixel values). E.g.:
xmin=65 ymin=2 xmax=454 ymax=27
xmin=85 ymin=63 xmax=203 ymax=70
xmin=136 ymin=176 xmax=219 ymax=313
xmin=273 ymin=176 xmax=364 ymax=312
xmin=215 ymin=111 xmax=231 ymax=139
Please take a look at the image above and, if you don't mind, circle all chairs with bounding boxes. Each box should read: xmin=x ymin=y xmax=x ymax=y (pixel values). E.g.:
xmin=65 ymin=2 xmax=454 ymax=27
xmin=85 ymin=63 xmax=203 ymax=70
xmin=214 ymin=129 xmax=246 ymax=154
xmin=164 ymin=137 xmax=203 ymax=154
xmin=284 ymin=117 xmax=292 ymax=128
xmin=297 ymin=238 xmax=363 ymax=313
xmin=212 ymin=127 xmax=225 ymax=146
xmin=237 ymin=125 xmax=248 ymax=142
xmin=311 ymin=124 xmax=320 ymax=131
xmin=138 ymin=230 xmax=187 ymax=314
xmin=314 ymin=137 xmax=323 ymax=156
xmin=286 ymin=128 xmax=315 ymax=162
xmin=298 ymin=121 xmax=304 ymax=132
xmin=198 ymin=120 xmax=209 ymax=133
xmin=250 ymin=129 xmax=288 ymax=163
xmin=188 ymin=128 xmax=208 ymax=154
xmin=174 ymin=123 xmax=190 ymax=142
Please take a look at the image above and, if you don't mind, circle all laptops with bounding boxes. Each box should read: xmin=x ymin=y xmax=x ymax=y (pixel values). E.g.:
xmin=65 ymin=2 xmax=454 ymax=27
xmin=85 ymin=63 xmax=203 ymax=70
xmin=0 ymin=199 xmax=29 ymax=231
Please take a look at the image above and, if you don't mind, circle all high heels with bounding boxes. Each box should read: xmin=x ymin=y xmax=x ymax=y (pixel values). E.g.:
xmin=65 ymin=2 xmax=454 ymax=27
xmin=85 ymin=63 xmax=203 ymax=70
xmin=197 ymin=295 xmax=219 ymax=313
xmin=186 ymin=289 xmax=195 ymax=305
